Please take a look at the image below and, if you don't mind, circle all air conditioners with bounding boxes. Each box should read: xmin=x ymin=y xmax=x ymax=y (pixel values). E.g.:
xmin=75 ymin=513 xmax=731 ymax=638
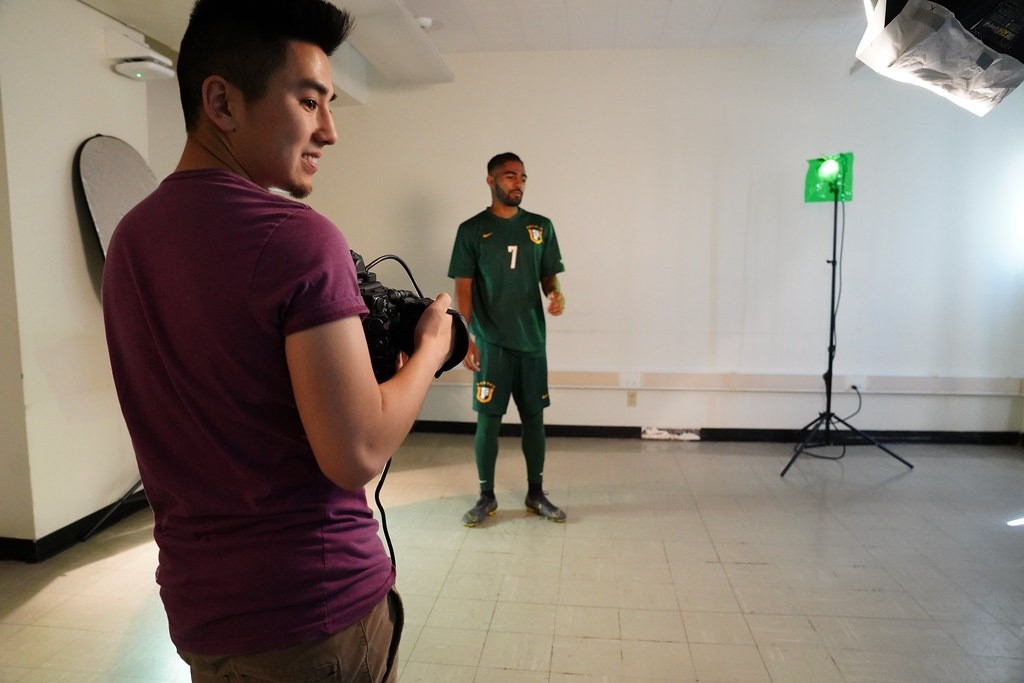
xmin=107 ymin=29 xmax=175 ymax=81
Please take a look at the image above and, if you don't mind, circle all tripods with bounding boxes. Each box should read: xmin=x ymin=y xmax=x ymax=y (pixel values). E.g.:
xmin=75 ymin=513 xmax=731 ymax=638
xmin=781 ymin=190 xmax=916 ymax=477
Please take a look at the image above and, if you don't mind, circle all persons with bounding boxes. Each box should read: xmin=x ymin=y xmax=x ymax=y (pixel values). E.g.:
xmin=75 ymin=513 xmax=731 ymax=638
xmin=448 ymin=153 xmax=568 ymax=526
xmin=98 ymin=1 xmax=455 ymax=683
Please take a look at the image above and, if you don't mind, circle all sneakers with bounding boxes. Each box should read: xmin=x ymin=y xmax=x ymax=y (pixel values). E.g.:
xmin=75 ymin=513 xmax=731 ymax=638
xmin=461 ymin=498 xmax=498 ymax=527
xmin=524 ymin=494 xmax=567 ymax=523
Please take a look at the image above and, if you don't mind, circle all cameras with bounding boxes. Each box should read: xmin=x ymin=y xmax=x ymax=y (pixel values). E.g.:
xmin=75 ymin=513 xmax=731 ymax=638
xmin=350 ymin=250 xmax=464 ymax=381
